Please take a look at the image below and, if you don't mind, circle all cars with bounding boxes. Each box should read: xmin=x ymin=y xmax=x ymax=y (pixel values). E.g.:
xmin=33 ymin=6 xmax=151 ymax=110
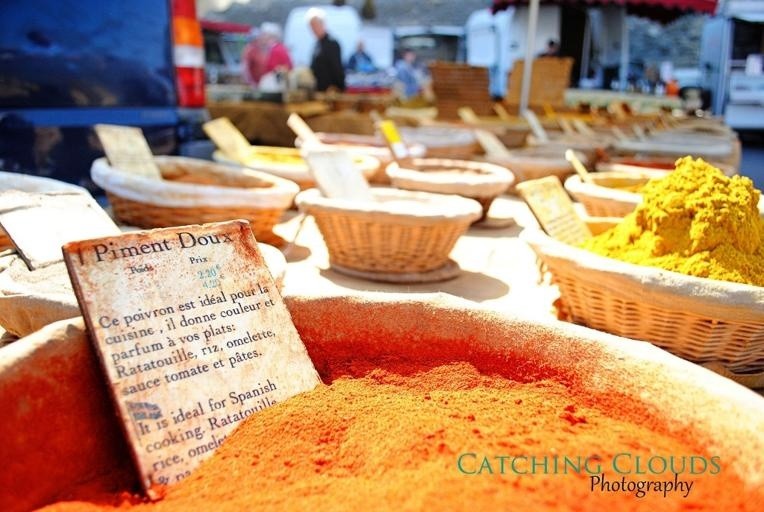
xmin=0 ymin=1 xmax=216 ymax=206
xmin=202 ymin=33 xmax=258 ymax=83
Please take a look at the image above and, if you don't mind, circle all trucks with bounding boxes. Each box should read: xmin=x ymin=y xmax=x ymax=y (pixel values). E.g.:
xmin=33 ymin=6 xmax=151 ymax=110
xmin=700 ymin=0 xmax=764 ymax=130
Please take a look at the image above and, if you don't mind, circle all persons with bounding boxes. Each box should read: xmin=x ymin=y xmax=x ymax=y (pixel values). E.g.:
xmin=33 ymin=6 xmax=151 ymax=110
xmin=634 ymin=65 xmax=668 ymax=94
xmin=309 ymin=15 xmax=346 ymax=89
xmin=242 ymin=22 xmax=294 ymax=105
xmin=395 ymin=46 xmax=420 ymax=93
xmin=349 ymin=40 xmax=371 ymax=70
xmin=540 ymin=40 xmax=560 ymax=57
